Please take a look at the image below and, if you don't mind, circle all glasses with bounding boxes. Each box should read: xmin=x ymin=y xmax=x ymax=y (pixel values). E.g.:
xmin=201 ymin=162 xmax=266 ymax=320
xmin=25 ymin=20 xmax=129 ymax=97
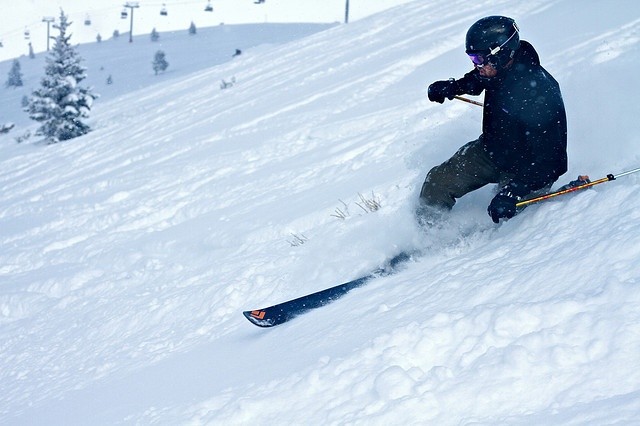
xmin=466 ymin=30 xmax=517 ymax=67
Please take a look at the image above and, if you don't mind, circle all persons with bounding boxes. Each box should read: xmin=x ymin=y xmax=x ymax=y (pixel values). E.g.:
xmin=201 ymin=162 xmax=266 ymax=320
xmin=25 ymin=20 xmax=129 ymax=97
xmin=415 ymin=16 xmax=568 ymax=228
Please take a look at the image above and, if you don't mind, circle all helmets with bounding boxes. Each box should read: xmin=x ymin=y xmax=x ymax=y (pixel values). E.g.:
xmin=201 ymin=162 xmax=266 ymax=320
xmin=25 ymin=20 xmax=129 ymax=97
xmin=466 ymin=15 xmax=520 ymax=66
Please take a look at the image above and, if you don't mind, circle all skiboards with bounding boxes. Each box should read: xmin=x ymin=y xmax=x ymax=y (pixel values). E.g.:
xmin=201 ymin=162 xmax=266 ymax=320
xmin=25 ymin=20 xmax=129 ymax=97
xmin=243 ymin=175 xmax=592 ymax=328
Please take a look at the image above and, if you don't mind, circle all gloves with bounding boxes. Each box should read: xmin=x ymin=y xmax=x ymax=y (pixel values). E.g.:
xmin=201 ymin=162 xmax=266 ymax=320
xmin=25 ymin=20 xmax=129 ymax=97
xmin=427 ymin=78 xmax=461 ymax=103
xmin=487 ymin=177 xmax=530 ymax=223
